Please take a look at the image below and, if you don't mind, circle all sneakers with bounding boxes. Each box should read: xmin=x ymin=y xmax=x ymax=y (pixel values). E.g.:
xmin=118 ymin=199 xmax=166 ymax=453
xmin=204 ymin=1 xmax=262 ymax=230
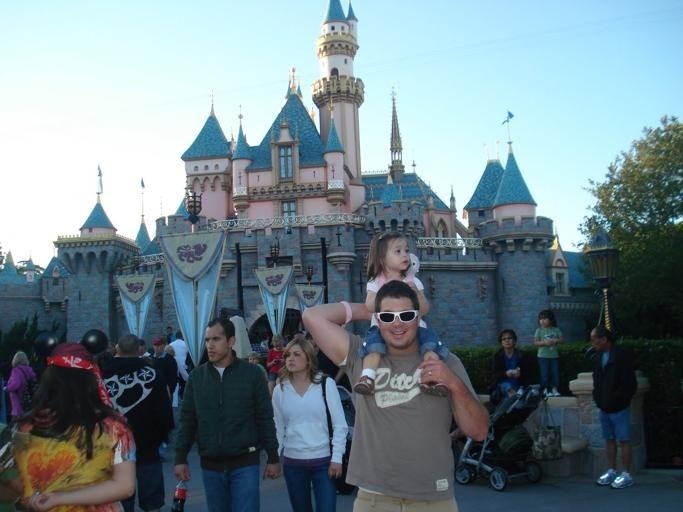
xmin=543 ymin=388 xmax=553 ymax=397
xmin=596 ymin=469 xmax=621 ymax=486
xmin=610 ymin=474 xmax=636 ymax=490
xmin=552 ymin=386 xmax=561 ymax=397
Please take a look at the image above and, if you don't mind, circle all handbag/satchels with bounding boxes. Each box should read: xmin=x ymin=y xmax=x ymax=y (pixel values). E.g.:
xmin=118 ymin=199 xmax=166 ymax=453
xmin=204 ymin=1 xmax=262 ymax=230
xmin=329 ymin=438 xmax=359 ymax=497
xmin=530 ymin=425 xmax=563 ymax=461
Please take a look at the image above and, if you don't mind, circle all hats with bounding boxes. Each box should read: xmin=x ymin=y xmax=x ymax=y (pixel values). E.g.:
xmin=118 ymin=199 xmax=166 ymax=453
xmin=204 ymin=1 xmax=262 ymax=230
xmin=152 ymin=337 xmax=163 ymax=345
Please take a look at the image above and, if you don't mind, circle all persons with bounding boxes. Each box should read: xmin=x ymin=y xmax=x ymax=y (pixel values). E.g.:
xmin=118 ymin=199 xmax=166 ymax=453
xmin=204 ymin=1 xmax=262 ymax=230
xmin=535 ymin=311 xmax=564 ymax=396
xmin=490 ymin=330 xmax=536 ymax=404
xmin=9 ymin=342 xmax=137 ymax=512
xmin=137 ymin=318 xmax=353 ymax=512
xmin=4 ymin=351 xmax=36 ymax=421
xmin=591 ymin=327 xmax=638 ymax=489
xmin=100 ymin=335 xmax=175 ymax=512
xmin=351 ymin=230 xmax=449 ymax=399
xmin=300 ymin=282 xmax=490 ymax=511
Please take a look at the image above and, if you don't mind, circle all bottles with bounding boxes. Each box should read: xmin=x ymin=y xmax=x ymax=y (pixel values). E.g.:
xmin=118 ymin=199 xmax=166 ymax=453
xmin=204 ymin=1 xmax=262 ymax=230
xmin=516 ymin=385 xmax=525 ymax=398
xmin=170 ymin=479 xmax=188 ymax=512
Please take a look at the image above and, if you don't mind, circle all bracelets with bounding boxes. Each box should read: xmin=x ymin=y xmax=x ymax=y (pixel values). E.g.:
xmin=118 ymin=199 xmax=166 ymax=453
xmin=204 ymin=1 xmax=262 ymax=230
xmin=340 ymin=300 xmax=352 ymax=328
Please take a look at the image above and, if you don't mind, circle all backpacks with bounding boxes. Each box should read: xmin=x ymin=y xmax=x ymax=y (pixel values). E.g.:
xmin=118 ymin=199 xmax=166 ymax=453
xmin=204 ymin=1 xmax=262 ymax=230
xmin=20 ymin=381 xmax=42 ymax=411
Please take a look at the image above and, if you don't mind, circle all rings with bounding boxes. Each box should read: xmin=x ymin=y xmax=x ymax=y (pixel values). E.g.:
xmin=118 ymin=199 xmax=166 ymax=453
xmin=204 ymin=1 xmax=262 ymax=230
xmin=428 ymin=370 xmax=432 ymax=376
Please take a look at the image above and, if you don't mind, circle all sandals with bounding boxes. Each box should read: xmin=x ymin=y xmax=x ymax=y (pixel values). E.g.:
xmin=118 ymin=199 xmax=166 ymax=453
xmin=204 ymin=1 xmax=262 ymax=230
xmin=353 ymin=375 xmax=375 ymax=396
xmin=418 ymin=379 xmax=448 ymax=398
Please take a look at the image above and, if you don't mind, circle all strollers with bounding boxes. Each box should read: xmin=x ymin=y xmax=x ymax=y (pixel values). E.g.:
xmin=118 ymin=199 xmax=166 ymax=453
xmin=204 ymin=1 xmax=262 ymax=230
xmin=455 ymin=381 xmax=548 ymax=490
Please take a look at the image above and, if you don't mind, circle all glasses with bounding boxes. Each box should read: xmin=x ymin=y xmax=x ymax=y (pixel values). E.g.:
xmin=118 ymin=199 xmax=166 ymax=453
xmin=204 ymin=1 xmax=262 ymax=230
xmin=376 ymin=310 xmax=419 ymax=323
xmin=501 ymin=336 xmax=513 ymax=340
